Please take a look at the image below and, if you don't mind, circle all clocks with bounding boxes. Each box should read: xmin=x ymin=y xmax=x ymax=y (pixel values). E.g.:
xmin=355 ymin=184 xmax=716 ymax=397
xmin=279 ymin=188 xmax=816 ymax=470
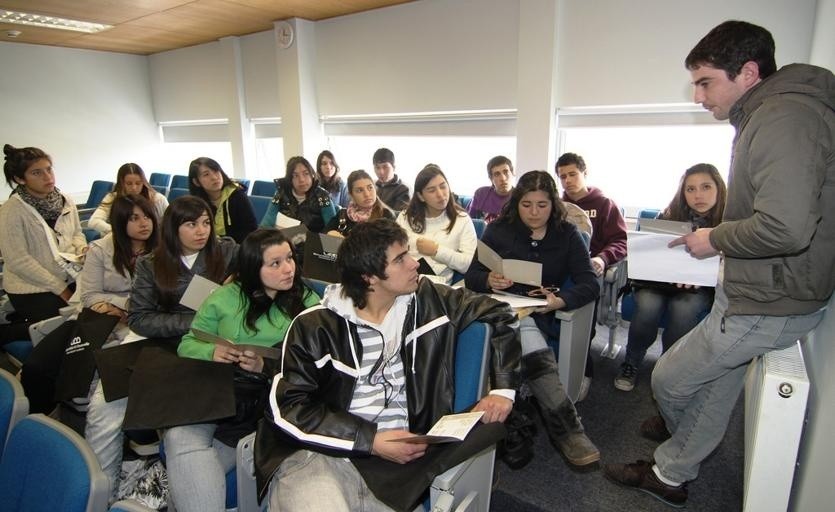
xmin=274 ymin=20 xmax=293 ymax=49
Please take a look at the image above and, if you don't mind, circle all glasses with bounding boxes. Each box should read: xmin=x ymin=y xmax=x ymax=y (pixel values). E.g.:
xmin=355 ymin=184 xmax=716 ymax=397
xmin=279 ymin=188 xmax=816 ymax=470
xmin=528 ymin=287 xmax=560 ymax=297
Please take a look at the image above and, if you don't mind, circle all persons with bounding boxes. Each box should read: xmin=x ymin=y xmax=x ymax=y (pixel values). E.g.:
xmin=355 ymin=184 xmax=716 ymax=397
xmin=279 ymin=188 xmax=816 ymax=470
xmin=165 ymin=231 xmax=322 ymax=512
xmin=604 ymin=20 xmax=835 ymax=507
xmin=614 ymin=164 xmax=727 ymax=390
xmin=465 ymin=171 xmax=602 ymax=467
xmin=0 ymin=146 xmax=515 ymax=418
xmin=85 ymin=196 xmax=240 ymax=503
xmin=554 ymin=152 xmax=627 ymax=401
xmin=254 ymin=217 xmax=521 ymax=512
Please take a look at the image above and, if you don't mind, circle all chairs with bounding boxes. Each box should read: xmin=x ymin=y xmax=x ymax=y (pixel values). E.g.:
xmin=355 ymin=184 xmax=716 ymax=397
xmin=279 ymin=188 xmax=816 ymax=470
xmin=0 ymin=172 xmax=668 ymax=512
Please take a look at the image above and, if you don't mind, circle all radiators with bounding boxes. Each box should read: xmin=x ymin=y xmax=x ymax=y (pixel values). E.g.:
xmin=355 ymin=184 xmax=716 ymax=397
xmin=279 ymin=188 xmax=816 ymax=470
xmin=743 ymin=340 xmax=811 ymax=512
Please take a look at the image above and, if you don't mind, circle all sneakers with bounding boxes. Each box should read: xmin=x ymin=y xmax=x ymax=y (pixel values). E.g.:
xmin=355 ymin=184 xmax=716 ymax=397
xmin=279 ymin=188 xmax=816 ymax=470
xmin=554 ymin=433 xmax=601 ymax=467
xmin=613 ymin=362 xmax=634 ymax=391
xmin=640 ymin=416 xmax=668 ymax=441
xmin=605 ymin=461 xmax=687 ymax=507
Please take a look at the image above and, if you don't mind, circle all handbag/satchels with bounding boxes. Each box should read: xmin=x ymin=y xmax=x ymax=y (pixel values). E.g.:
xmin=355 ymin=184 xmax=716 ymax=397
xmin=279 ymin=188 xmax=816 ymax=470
xmin=53 ymin=307 xmax=117 ymax=399
xmin=497 ymin=397 xmax=538 ymax=469
xmin=66 ymin=262 xmax=82 ymax=277
xmin=119 ymin=456 xmax=169 ymax=510
xmin=215 ymin=374 xmax=270 ymax=447
